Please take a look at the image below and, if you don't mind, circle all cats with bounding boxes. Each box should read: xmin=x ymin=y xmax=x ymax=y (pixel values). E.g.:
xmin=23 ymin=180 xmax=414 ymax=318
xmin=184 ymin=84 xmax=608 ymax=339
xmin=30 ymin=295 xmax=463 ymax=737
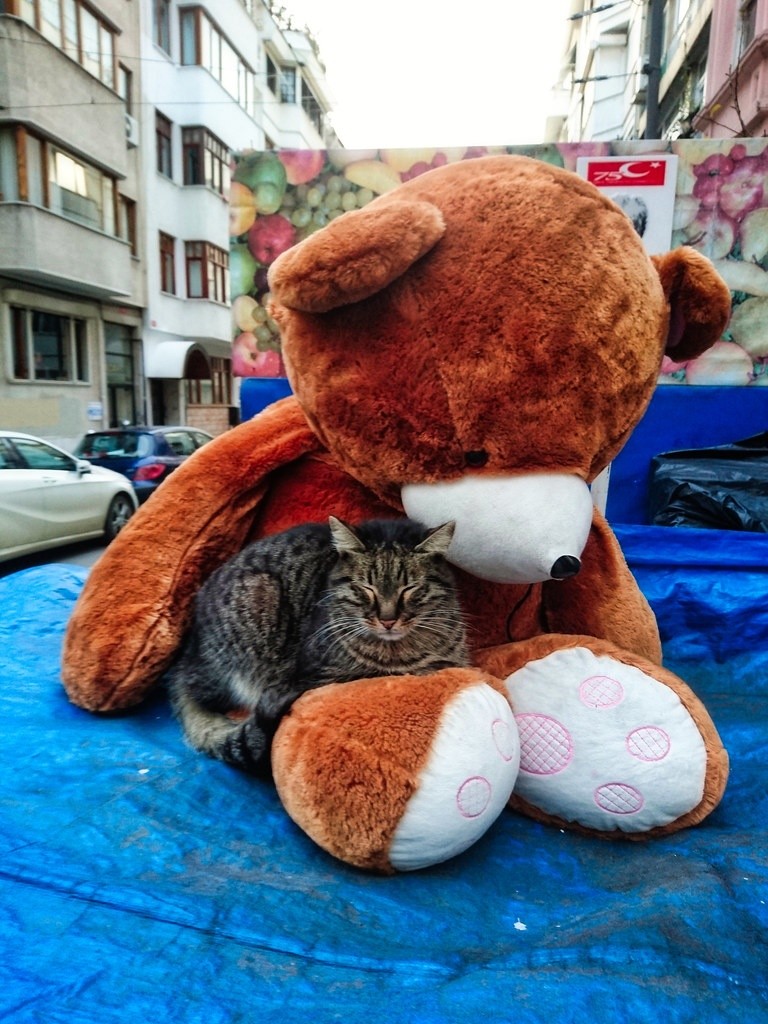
xmin=168 ymin=515 xmax=471 ymax=763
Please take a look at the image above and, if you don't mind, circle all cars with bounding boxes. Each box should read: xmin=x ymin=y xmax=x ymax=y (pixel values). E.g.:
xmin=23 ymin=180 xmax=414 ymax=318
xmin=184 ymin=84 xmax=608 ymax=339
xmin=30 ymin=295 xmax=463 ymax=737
xmin=71 ymin=426 xmax=215 ymax=505
xmin=1 ymin=430 xmax=141 ymax=565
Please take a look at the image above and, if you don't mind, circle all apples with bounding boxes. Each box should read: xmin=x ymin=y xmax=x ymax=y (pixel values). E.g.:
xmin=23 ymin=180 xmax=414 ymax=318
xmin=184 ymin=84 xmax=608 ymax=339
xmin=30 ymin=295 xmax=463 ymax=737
xmin=225 ymin=139 xmax=768 ymax=384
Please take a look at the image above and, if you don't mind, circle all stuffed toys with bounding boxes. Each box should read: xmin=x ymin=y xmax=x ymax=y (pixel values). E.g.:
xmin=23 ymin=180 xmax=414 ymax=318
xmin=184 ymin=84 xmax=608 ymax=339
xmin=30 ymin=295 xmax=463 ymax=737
xmin=60 ymin=154 xmax=737 ymax=875
